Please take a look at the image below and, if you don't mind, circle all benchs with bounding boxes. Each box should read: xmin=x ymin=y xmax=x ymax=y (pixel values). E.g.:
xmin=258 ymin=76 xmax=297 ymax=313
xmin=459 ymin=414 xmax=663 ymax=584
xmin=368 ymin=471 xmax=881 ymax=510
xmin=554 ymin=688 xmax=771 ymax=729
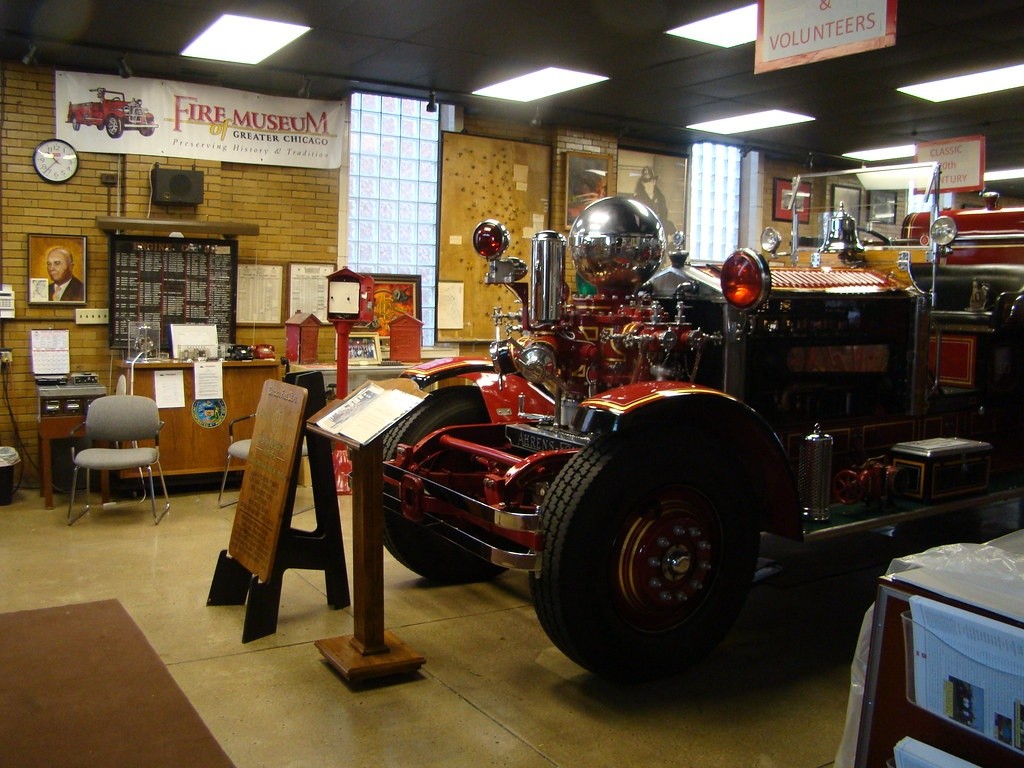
xmin=897 ymin=254 xmax=1024 ymax=331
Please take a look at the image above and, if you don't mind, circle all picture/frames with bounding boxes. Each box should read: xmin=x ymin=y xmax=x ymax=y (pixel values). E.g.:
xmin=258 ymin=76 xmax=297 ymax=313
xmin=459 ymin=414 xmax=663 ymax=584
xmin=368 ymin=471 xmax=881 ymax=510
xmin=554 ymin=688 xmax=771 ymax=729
xmin=27 ymin=234 xmax=88 ymax=305
xmin=832 ymin=184 xmax=862 ymax=225
xmin=772 ymin=178 xmax=813 ymax=225
xmin=235 ymin=259 xmax=287 ymax=326
xmin=564 ymin=152 xmax=612 ymax=230
xmin=617 ymin=146 xmax=691 ymax=252
xmin=347 ymin=274 xmax=421 ymax=340
xmin=289 ymin=261 xmax=339 ymax=325
xmin=865 ymin=190 xmax=897 ymax=224
xmin=349 ymin=331 xmax=381 ymax=365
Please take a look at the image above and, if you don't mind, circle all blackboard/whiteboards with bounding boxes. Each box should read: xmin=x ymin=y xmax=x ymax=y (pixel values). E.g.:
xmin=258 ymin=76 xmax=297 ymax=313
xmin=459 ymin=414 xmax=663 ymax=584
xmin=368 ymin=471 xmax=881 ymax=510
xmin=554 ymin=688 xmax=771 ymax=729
xmin=109 ymin=234 xmax=238 ymax=351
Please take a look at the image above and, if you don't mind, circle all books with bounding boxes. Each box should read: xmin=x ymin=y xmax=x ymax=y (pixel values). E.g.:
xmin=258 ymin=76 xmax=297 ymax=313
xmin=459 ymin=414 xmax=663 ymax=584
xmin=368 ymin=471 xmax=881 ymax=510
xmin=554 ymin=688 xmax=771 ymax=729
xmin=893 ymin=736 xmax=981 ymax=768
xmin=317 ymin=382 xmax=423 ymax=445
xmin=909 ymin=595 xmax=1024 ymax=756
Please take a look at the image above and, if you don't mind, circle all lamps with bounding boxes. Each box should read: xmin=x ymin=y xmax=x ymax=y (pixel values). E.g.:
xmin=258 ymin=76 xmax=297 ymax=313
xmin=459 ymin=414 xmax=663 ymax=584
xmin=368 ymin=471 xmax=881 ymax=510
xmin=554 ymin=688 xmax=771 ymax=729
xmin=180 ymin=4 xmax=313 ymax=65
xmin=739 ymin=144 xmax=755 ymax=158
xmin=426 ymin=90 xmax=438 ymax=112
xmin=529 ymin=103 xmax=544 ymax=128
xmin=802 ymin=152 xmax=815 ymax=169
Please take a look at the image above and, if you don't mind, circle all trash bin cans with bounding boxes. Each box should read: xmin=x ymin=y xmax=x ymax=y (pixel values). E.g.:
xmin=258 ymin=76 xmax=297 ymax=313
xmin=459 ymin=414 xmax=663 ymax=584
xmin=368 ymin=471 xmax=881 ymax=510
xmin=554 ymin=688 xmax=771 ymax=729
xmin=0 ymin=446 xmax=19 ymax=506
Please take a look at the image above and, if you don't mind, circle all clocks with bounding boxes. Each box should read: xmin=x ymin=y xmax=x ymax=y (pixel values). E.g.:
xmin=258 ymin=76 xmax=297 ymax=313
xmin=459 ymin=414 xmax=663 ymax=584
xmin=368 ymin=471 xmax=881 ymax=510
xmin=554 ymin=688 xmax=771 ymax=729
xmin=33 ymin=140 xmax=80 ymax=182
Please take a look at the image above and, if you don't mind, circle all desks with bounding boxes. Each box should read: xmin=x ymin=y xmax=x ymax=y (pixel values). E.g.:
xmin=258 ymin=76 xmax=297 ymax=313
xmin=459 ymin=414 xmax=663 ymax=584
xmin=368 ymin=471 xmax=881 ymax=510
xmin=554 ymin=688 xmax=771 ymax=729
xmin=116 ymin=357 xmax=281 ymax=479
xmin=39 ymin=414 xmax=111 ymax=509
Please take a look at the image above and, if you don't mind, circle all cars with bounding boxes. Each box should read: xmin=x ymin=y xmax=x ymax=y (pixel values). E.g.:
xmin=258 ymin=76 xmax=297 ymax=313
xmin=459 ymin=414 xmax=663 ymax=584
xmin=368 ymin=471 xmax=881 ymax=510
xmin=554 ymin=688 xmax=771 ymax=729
xmin=65 ymin=88 xmax=159 ymax=139
xmin=379 ymin=161 xmax=1024 ymax=685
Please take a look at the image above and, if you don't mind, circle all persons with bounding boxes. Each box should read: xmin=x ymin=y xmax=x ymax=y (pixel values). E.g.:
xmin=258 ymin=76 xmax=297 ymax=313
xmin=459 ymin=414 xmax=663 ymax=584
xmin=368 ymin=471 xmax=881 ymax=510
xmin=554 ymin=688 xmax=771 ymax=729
xmin=349 ymin=344 xmax=374 ymax=358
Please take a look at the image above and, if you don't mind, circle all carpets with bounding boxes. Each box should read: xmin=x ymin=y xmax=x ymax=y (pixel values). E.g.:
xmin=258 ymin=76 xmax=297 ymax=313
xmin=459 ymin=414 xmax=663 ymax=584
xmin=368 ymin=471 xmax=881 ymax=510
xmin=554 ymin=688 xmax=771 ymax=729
xmin=0 ymin=598 xmax=240 ymax=768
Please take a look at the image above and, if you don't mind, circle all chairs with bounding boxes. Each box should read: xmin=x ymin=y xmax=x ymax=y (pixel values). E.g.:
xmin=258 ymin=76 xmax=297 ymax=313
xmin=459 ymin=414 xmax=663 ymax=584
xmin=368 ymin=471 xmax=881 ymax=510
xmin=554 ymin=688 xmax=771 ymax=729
xmin=65 ymin=394 xmax=170 ymax=527
xmin=218 ymin=383 xmax=314 ymax=516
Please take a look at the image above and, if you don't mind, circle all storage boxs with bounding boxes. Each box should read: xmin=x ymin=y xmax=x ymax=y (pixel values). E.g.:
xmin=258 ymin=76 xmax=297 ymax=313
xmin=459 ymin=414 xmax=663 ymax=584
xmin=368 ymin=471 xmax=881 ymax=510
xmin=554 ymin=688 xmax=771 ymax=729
xmin=890 ymin=439 xmax=993 ymax=502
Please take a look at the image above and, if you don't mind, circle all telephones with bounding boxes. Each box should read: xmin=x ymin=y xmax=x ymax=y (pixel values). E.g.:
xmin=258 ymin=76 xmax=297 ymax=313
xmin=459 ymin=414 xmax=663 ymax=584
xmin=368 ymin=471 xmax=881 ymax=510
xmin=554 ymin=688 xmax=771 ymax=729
xmin=228 ymin=344 xmax=253 ymax=361
xmin=251 ymin=344 xmax=276 ymax=359
